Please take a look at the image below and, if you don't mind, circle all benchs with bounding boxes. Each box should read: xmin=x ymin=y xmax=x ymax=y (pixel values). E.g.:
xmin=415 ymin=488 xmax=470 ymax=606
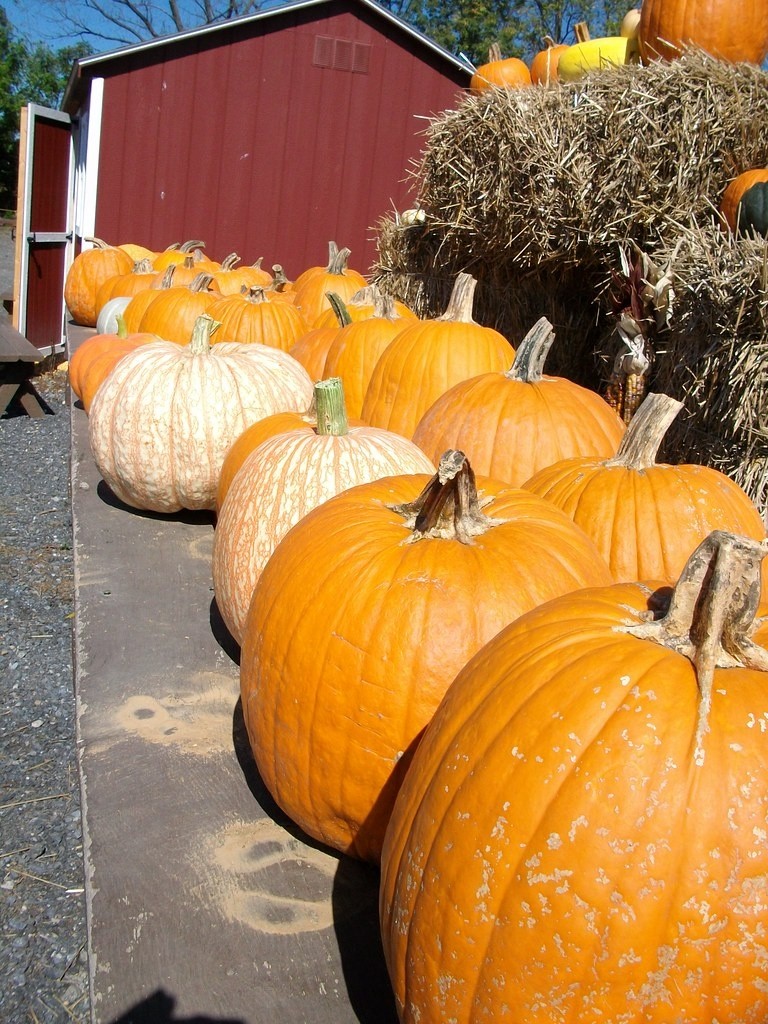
xmin=0 ymin=307 xmax=49 ymax=418
